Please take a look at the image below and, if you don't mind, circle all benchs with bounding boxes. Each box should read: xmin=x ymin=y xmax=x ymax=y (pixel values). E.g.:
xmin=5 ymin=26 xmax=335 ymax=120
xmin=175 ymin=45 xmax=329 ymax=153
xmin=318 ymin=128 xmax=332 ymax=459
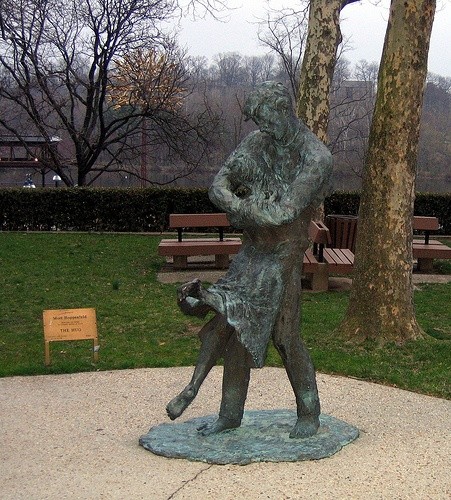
xmin=158 ymin=214 xmax=241 ymax=267
xmin=408 ymin=217 xmax=451 ymax=272
xmin=303 ymin=221 xmax=355 ymax=291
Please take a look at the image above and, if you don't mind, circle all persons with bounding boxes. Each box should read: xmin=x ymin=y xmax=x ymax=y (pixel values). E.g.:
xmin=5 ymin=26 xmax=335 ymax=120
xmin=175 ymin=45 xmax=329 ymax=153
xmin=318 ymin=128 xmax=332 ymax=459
xmin=165 ymin=81 xmax=334 ymax=439
